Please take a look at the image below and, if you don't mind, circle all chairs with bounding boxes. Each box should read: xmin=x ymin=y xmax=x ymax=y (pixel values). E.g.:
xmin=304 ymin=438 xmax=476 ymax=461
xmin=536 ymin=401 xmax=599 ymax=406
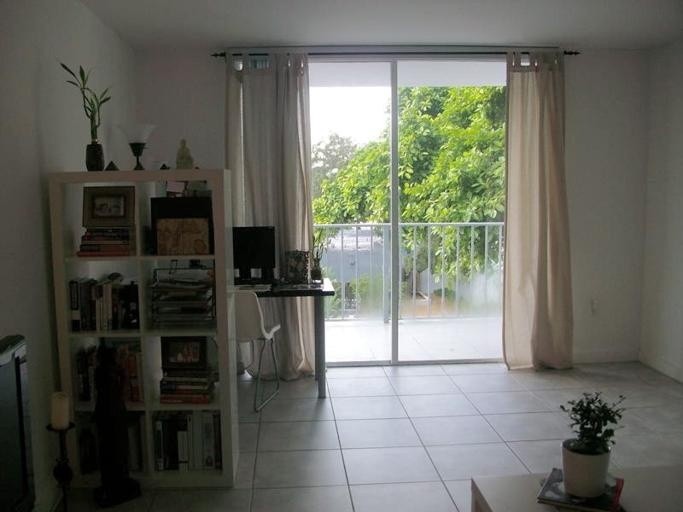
xmin=235 ymin=290 xmax=281 ymax=411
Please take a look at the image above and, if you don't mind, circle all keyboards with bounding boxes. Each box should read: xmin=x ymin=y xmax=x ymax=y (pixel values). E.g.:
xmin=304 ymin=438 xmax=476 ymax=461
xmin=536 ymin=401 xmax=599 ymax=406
xmin=234 ymin=284 xmax=272 ymax=293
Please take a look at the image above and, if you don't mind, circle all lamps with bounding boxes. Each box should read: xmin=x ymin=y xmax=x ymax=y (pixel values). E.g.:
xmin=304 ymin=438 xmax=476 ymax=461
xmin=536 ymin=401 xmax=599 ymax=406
xmin=117 ymin=122 xmax=157 ymax=171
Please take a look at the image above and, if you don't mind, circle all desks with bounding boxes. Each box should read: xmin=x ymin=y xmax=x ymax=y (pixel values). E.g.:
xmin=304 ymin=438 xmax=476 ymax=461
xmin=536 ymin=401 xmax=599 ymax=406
xmin=471 ymin=465 xmax=683 ymax=512
xmin=235 ymin=277 xmax=335 ymax=397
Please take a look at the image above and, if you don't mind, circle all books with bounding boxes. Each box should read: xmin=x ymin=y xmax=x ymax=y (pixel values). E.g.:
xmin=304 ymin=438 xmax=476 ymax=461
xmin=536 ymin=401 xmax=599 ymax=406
xmin=69 ymin=225 xmax=222 ymax=472
xmin=537 ymin=467 xmax=626 ymax=512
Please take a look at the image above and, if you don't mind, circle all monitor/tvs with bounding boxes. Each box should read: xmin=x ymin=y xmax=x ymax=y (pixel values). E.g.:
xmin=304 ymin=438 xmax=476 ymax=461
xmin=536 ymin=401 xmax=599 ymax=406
xmin=0 ymin=336 xmax=36 ymax=512
xmin=232 ymin=226 xmax=275 ymax=283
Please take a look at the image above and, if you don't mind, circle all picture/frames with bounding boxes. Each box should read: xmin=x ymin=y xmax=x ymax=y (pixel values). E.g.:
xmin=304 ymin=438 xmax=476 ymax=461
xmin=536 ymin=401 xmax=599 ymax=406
xmin=83 ymin=186 xmax=135 ymax=227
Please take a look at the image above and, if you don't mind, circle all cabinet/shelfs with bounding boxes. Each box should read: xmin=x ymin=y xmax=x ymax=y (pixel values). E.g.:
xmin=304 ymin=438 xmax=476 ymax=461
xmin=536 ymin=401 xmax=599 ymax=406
xmin=44 ymin=169 xmax=238 ymax=488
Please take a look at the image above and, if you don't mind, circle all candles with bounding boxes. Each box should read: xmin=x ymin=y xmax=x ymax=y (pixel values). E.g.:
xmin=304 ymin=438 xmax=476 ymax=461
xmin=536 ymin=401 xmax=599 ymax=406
xmin=51 ymin=391 xmax=70 ymax=429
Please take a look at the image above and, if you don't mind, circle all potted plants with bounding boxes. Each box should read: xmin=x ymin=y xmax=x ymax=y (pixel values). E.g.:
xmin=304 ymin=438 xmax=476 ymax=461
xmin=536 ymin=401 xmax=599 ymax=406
xmin=560 ymin=392 xmax=629 ymax=498
xmin=60 ymin=60 xmax=114 ymax=170
